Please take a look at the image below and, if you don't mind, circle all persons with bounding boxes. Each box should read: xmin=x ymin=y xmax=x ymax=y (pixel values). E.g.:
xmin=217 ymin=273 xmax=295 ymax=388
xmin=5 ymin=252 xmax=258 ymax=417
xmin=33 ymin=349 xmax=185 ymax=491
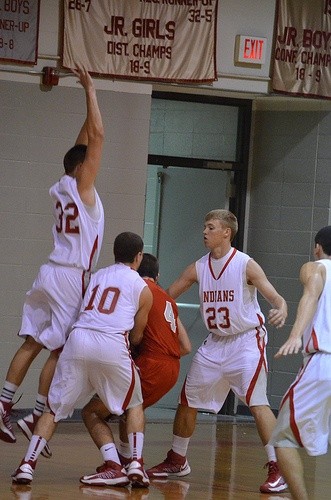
xmin=11 ymin=232 xmax=152 ymax=489
xmin=147 ymin=209 xmax=287 ymax=492
xmin=0 ymin=63 xmax=105 ymax=458
xmin=269 ymin=227 xmax=331 ymax=500
xmin=80 ymin=253 xmax=191 ymax=485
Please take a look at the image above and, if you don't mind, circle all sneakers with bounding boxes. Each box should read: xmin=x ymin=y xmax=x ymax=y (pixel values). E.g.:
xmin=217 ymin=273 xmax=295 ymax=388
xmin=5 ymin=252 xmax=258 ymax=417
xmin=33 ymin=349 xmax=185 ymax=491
xmin=259 ymin=461 xmax=288 ymax=492
xmin=10 ymin=484 xmax=32 ymax=500
xmin=17 ymin=411 xmax=52 ymax=458
xmin=260 ymin=493 xmax=293 ymax=500
xmin=130 ymin=486 xmax=150 ymax=500
xmin=11 ymin=457 xmax=37 ymax=483
xmin=79 ymin=460 xmax=131 ymax=486
xmin=0 ymin=392 xmax=23 ymax=443
xmin=150 ymin=479 xmax=190 ymax=498
xmin=78 ymin=485 xmax=129 ymax=500
xmin=127 ymin=457 xmax=150 ymax=488
xmin=145 ymin=449 xmax=192 ymax=477
xmin=96 ymin=449 xmax=133 ymax=473
